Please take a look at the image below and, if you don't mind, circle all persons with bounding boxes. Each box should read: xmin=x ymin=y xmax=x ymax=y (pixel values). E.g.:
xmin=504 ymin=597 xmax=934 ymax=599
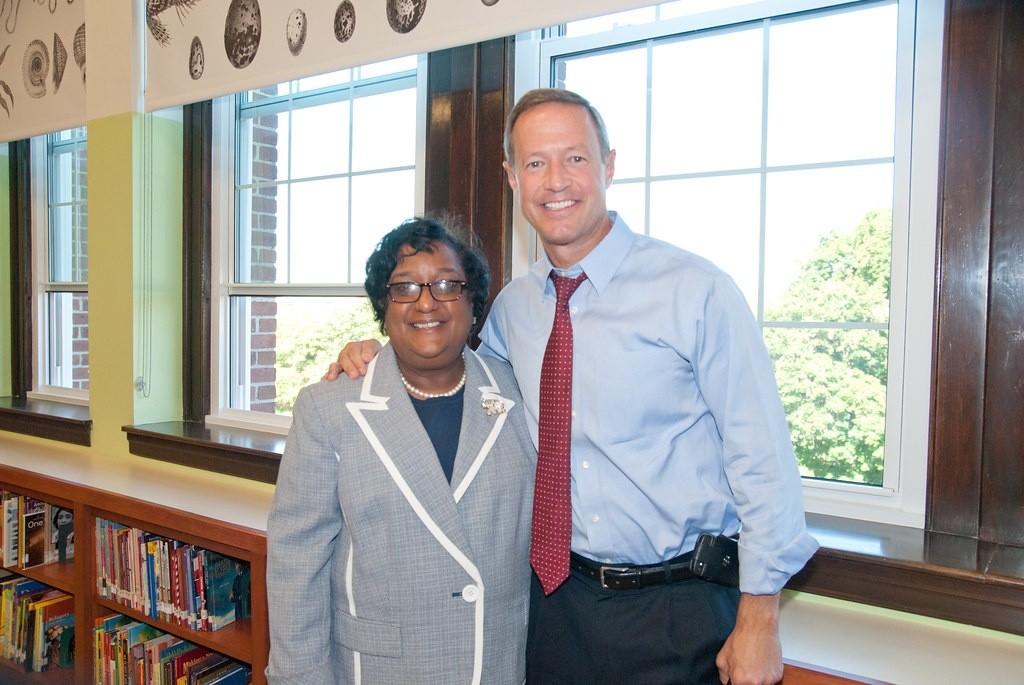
xmin=50 ymin=509 xmax=74 ymax=549
xmin=320 ymin=88 xmax=822 ymax=685
xmin=265 ymin=206 xmax=539 ymax=685
xmin=229 ymin=562 xmax=251 ymax=620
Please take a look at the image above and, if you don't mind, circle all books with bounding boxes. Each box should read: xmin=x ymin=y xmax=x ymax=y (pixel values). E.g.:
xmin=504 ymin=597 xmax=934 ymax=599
xmin=94 ymin=518 xmax=252 ymax=632
xmin=0 ymin=576 xmax=73 ymax=674
xmin=0 ymin=490 xmax=75 ymax=571
xmin=93 ymin=613 xmax=252 ymax=685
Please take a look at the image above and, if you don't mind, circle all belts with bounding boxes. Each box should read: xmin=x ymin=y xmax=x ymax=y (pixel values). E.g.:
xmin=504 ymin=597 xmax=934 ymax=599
xmin=566 ymin=547 xmax=695 ymax=593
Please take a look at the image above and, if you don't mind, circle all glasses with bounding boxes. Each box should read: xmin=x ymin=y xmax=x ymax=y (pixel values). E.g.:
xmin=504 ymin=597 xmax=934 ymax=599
xmin=386 ymin=280 xmax=472 ymax=303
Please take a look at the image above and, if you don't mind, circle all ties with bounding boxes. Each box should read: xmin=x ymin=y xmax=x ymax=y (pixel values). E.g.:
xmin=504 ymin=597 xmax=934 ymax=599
xmin=529 ymin=269 xmax=587 ymax=596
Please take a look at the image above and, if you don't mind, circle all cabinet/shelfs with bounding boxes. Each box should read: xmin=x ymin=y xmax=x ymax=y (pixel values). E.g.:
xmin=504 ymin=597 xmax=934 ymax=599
xmin=0 ymin=459 xmax=268 ymax=685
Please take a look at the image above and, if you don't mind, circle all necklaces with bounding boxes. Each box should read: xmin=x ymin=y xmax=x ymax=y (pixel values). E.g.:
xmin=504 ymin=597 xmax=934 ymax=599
xmin=396 ymin=353 xmax=467 ymax=398
